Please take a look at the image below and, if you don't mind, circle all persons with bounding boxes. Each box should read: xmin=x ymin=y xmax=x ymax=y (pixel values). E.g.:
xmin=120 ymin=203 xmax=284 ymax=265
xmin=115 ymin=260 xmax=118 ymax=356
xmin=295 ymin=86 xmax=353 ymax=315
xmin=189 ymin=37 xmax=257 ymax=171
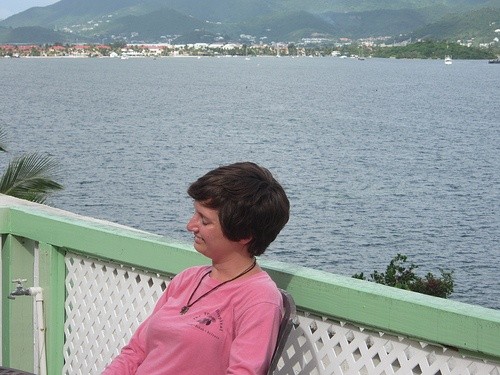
xmin=97 ymin=161 xmax=291 ymax=375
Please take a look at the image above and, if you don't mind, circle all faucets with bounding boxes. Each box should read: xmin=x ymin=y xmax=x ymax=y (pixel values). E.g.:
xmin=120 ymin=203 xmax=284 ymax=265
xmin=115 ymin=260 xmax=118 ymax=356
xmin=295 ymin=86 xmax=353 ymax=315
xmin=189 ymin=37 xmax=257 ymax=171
xmin=6 ymin=277 xmax=29 ymax=300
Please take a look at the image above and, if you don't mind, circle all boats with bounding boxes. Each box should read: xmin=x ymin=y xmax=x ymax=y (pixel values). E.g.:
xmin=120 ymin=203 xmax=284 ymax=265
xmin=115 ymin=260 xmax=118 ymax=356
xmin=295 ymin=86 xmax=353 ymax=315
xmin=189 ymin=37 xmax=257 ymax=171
xmin=445 ymin=57 xmax=452 ymax=64
xmin=488 ymin=59 xmax=500 ymax=64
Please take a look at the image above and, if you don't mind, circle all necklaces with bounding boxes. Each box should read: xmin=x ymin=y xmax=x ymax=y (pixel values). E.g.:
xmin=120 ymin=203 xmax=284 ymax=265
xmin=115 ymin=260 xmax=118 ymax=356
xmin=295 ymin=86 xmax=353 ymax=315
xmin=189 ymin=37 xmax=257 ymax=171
xmin=180 ymin=258 xmax=257 ymax=314
